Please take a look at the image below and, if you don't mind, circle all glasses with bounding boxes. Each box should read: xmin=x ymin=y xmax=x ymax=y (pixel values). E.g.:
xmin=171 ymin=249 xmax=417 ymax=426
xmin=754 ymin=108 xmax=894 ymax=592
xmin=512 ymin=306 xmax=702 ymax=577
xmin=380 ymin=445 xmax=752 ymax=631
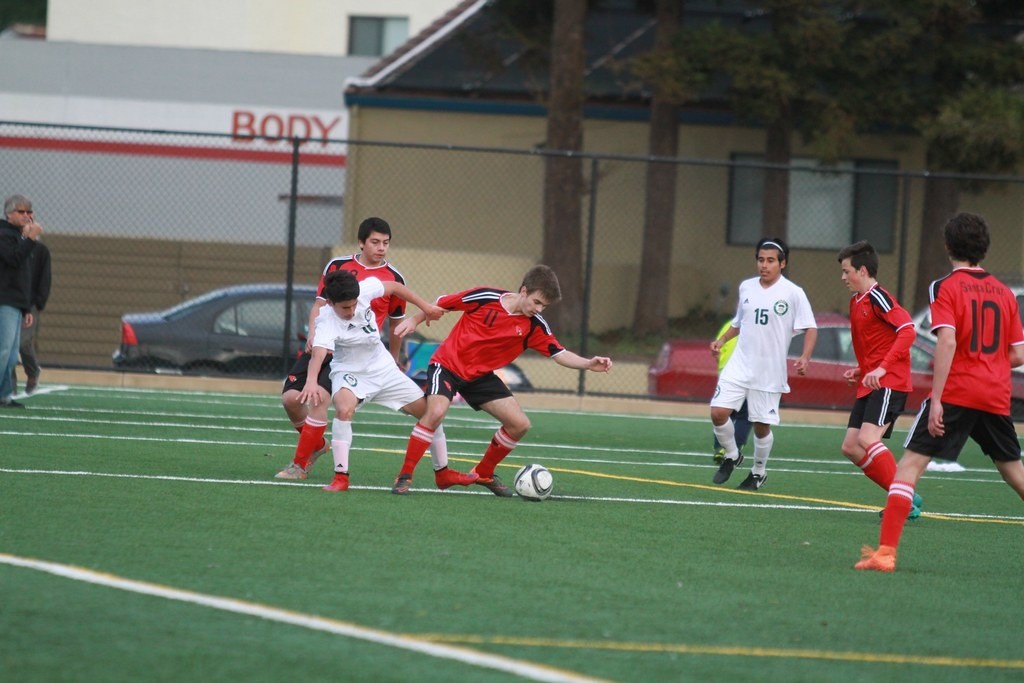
xmin=14 ymin=209 xmax=32 ymax=215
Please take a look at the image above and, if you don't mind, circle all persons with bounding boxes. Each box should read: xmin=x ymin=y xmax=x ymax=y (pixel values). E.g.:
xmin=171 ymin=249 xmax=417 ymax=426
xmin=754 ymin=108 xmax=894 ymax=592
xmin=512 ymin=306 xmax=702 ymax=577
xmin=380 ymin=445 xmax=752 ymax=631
xmin=838 ymin=240 xmax=924 ymax=520
xmin=392 ymin=264 xmax=612 ymax=497
xmin=295 ymin=270 xmax=479 ymax=491
xmin=0 ymin=194 xmax=51 ymax=409
xmin=853 ymin=212 xmax=1024 ymax=572
xmin=710 ymin=238 xmax=817 ymax=492
xmin=280 ymin=217 xmax=406 ymax=477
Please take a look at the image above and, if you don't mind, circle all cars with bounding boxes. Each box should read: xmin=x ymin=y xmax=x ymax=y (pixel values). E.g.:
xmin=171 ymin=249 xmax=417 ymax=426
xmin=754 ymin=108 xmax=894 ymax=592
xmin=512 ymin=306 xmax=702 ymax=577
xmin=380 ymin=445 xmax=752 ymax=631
xmin=109 ymin=283 xmax=534 ymax=390
xmin=644 ymin=285 xmax=1024 ymax=416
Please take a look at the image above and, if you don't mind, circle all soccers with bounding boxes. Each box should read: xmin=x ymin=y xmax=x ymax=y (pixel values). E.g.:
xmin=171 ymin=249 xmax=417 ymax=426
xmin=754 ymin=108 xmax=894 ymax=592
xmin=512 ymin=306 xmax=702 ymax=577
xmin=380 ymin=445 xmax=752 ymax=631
xmin=514 ymin=464 xmax=554 ymax=501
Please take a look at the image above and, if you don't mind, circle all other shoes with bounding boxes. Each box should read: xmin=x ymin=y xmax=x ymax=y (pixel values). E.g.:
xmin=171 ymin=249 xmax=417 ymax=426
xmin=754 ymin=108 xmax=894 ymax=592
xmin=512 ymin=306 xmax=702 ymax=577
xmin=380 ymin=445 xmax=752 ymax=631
xmin=0 ymin=399 xmax=26 ymax=408
xmin=713 ymin=448 xmax=725 ymax=463
xmin=25 ymin=367 xmax=41 ymax=394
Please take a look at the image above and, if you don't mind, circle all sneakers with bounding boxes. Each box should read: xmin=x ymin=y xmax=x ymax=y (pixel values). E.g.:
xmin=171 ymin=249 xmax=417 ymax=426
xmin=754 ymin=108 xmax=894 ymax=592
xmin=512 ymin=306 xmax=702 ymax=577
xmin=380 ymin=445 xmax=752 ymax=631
xmin=735 ymin=471 xmax=767 ymax=492
xmin=469 ymin=466 xmax=513 ymax=497
xmin=713 ymin=450 xmax=744 ymax=484
xmin=322 ymin=472 xmax=349 ymax=492
xmin=879 ymin=493 xmax=923 ymax=521
xmin=306 ymin=437 xmax=330 ymax=465
xmin=855 ymin=545 xmax=896 ymax=573
xmin=433 ymin=466 xmax=479 ymax=490
xmin=391 ymin=474 xmax=412 ymax=495
xmin=274 ymin=464 xmax=307 ymax=480
